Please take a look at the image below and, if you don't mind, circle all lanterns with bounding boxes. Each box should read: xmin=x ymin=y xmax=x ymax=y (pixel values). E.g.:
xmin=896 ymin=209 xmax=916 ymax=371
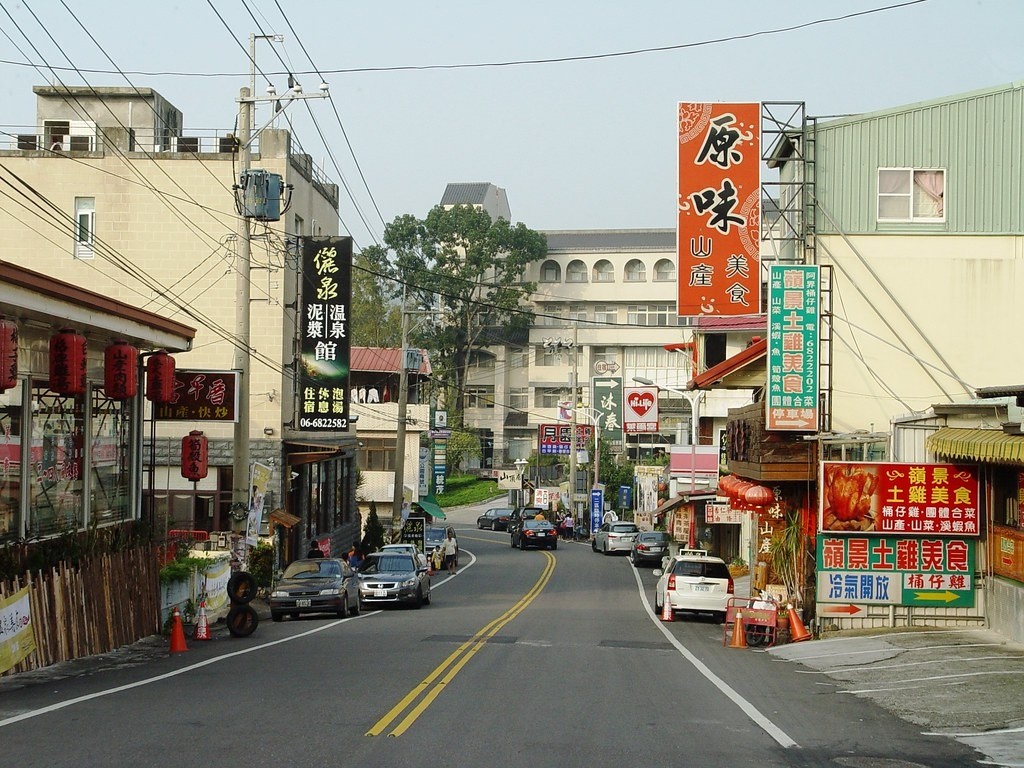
xmin=49 ymin=327 xmax=87 ymax=398
xmin=718 ymin=473 xmax=773 ymax=524
xmin=181 ymin=431 xmax=208 ymax=482
xmin=105 ymin=340 xmax=137 ymax=401
xmin=0 ymin=315 xmax=18 ymax=394
xmin=146 ymin=349 xmax=175 ymax=408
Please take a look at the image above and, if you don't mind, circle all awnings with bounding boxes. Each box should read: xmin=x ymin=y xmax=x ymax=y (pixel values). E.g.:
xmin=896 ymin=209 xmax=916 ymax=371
xmin=651 ymin=495 xmax=684 ymax=518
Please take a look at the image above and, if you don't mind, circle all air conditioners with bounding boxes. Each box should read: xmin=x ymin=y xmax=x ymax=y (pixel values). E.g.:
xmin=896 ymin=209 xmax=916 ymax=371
xmin=9 ymin=135 xmax=239 ymax=152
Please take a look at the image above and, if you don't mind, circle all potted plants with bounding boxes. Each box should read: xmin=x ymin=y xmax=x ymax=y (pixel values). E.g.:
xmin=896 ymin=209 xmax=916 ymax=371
xmin=769 ymin=509 xmax=804 ymax=629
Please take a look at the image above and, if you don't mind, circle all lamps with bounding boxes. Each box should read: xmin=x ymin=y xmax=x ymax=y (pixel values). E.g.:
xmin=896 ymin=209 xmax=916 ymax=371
xmin=264 ymin=427 xmax=274 ymax=435
xmin=349 ymin=414 xmax=359 ymax=423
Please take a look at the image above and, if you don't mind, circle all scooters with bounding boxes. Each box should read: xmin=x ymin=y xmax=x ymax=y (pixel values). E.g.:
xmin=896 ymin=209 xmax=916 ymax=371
xmin=744 ymin=588 xmax=781 ymax=647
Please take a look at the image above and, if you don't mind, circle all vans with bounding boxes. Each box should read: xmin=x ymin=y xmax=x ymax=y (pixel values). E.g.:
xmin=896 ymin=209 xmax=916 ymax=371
xmin=507 ymin=507 xmax=542 ymax=532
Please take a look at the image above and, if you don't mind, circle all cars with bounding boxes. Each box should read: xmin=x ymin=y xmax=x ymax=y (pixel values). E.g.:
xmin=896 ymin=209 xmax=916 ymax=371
xmin=477 ymin=508 xmax=514 ymax=531
xmin=270 ymin=558 xmax=360 ymax=622
xmin=356 ymin=549 xmax=432 ymax=608
xmin=379 ymin=544 xmax=427 ymax=570
xmin=630 ymin=532 xmax=672 ymax=567
xmin=510 ymin=519 xmax=557 ymax=550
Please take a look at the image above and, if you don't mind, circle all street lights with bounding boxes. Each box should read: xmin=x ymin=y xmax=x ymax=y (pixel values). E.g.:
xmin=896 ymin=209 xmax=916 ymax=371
xmin=633 ymin=377 xmax=706 ymax=550
xmin=557 ymin=405 xmax=604 ymax=489
xmin=514 ymin=459 xmax=528 ymax=507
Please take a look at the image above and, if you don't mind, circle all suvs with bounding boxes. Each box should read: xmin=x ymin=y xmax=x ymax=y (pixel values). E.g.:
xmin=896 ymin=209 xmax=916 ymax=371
xmin=652 ymin=556 xmax=734 ymax=622
xmin=592 ymin=521 xmax=639 ymax=555
xmin=425 ymin=527 xmax=459 ymax=569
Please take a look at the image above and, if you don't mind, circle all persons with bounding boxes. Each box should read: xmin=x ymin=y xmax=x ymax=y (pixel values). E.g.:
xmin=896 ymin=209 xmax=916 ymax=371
xmin=556 ymin=509 xmax=588 ymax=543
xmin=431 ymin=545 xmax=444 ymax=575
xmin=442 ymin=531 xmax=458 ymax=575
xmin=342 ymin=542 xmax=364 ymax=571
xmin=534 ymin=511 xmax=545 ymax=520
xmin=307 ymin=541 xmax=324 ymax=558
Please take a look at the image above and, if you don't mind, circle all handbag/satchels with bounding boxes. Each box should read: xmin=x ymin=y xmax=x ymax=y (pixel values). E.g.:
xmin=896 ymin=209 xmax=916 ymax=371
xmin=560 ymin=523 xmax=566 ymax=529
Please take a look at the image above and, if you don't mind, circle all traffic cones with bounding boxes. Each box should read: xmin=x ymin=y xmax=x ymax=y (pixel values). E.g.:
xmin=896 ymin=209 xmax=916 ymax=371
xmin=785 ymin=602 xmax=811 ymax=642
xmin=193 ymin=602 xmax=211 ymax=641
xmin=166 ymin=607 xmax=190 ymax=652
xmin=727 ymin=607 xmax=749 ymax=649
xmin=660 ymin=592 xmax=675 ymax=622
